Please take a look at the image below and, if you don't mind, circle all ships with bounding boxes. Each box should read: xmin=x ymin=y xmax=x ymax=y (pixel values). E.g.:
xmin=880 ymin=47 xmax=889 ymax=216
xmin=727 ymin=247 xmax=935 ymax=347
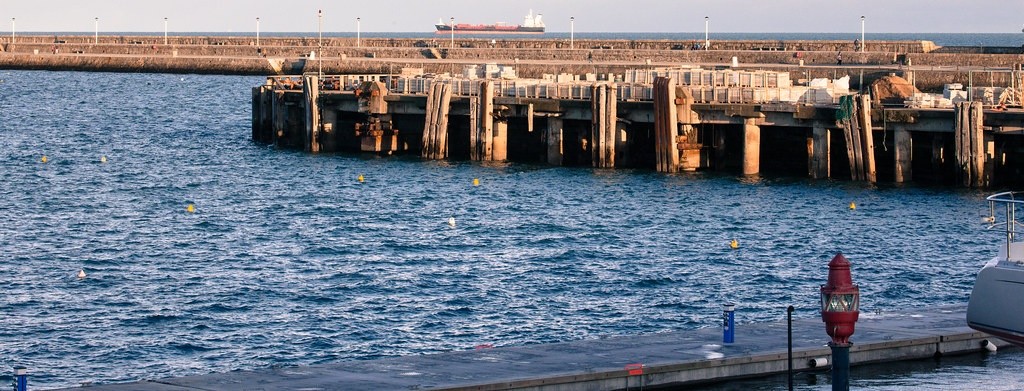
xmin=435 ymin=8 xmax=545 ymax=34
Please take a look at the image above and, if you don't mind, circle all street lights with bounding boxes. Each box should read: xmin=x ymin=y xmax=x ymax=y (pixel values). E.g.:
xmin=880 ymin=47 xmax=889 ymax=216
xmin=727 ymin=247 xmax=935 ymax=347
xmin=570 ymin=17 xmax=574 ymax=49
xmin=12 ymin=18 xmax=15 ymax=42
xmin=820 ymin=251 xmax=859 ymax=391
xmin=256 ymin=18 xmax=259 ymax=44
xmin=318 ymin=10 xmax=322 ymax=78
xmin=164 ymin=17 xmax=168 ymax=44
xmin=705 ymin=16 xmax=709 ymax=50
xmin=357 ymin=18 xmax=360 ymax=47
xmin=95 ymin=17 xmax=98 ymax=44
xmin=861 ymin=16 xmax=866 ymax=53
xmin=451 ymin=17 xmax=454 ymax=48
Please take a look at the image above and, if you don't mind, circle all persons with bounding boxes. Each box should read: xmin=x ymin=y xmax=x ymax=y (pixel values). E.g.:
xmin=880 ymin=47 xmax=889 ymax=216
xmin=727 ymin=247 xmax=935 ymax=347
xmin=837 ymin=52 xmax=842 ymax=65
xmin=491 ymin=37 xmax=496 ymax=46
xmin=854 ymin=39 xmax=859 ymax=52
xmin=587 ymin=52 xmax=594 ymax=63
xmin=691 ymin=41 xmax=700 ymax=50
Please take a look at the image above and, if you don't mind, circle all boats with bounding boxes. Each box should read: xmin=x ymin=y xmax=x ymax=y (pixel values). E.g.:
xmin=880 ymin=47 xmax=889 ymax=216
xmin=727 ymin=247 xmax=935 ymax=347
xmin=966 ymin=189 xmax=1024 ymax=347
